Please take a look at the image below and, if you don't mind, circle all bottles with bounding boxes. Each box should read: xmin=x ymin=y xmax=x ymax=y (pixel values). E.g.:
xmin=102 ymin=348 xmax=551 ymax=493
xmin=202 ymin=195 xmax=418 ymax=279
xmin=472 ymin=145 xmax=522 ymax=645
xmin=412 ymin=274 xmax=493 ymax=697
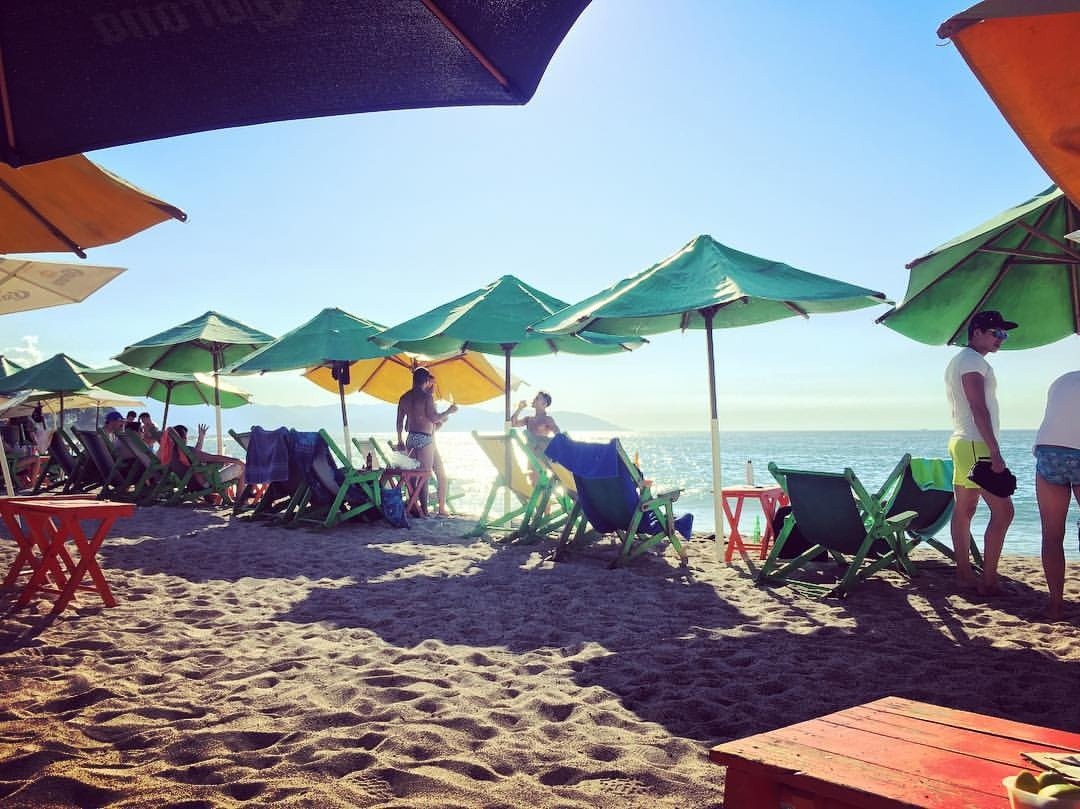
xmin=367 ymin=453 xmax=373 ymax=473
xmin=516 ymin=400 xmax=533 ymax=407
xmin=753 ymin=516 xmax=760 ymax=545
xmin=13 ymin=440 xmax=49 ymax=458
xmin=744 ymin=457 xmax=755 ymax=488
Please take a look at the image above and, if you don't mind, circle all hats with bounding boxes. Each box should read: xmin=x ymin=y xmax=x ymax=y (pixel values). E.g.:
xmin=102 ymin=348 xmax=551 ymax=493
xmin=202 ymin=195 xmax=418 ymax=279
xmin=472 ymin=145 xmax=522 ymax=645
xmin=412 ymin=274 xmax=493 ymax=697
xmin=971 ymin=311 xmax=1018 ymax=330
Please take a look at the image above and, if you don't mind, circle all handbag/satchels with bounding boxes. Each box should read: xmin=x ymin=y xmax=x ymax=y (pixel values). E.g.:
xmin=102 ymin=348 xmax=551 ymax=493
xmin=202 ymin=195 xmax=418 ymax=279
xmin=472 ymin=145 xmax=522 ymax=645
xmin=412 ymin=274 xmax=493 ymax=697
xmin=967 ymin=456 xmax=1017 ymax=496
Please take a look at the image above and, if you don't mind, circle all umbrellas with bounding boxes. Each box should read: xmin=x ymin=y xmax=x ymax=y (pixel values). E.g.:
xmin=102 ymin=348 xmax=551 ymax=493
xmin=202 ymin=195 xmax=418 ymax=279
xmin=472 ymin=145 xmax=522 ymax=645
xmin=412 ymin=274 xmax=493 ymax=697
xmin=0 ymin=353 xmax=146 ymax=427
xmin=213 ymin=306 xmax=403 ymax=470
xmin=76 ymin=362 xmax=255 ymax=434
xmin=526 ymin=234 xmax=895 ymax=565
xmin=110 ymin=311 xmax=278 ymax=454
xmin=0 ymin=152 xmax=189 ymax=259
xmin=0 ymin=0 xmax=592 ymax=164
xmin=0 ymin=256 xmax=128 ymax=313
xmin=299 ymin=349 xmax=530 ymax=407
xmin=369 ymin=275 xmax=651 ymax=537
xmin=874 ymin=198 xmax=1080 ymax=352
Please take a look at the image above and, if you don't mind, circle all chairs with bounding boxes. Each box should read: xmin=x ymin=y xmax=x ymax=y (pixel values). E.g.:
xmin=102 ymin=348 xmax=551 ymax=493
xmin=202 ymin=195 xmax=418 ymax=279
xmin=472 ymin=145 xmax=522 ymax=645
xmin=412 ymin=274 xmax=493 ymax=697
xmin=0 ymin=425 xmax=689 ymax=564
xmin=754 ymin=453 xmax=985 ymax=596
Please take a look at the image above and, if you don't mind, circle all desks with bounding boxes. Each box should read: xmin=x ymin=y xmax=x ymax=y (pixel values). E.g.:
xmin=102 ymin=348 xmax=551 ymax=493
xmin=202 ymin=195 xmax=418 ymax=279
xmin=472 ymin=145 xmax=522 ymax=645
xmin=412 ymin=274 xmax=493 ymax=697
xmin=382 ymin=467 xmax=433 ymax=519
xmin=0 ymin=494 xmax=96 ymax=601
xmin=6 ymin=500 xmax=136 ymax=613
xmin=722 ymin=485 xmax=790 ymax=561
xmin=709 ymin=696 xmax=1080 ymax=809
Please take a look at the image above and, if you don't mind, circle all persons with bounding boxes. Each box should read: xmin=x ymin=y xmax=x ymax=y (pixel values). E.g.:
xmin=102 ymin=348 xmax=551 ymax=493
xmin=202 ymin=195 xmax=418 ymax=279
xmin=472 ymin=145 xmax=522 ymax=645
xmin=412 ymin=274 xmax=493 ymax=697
xmin=944 ymin=311 xmax=1021 ymax=598
xmin=397 ymin=367 xmax=459 ymax=515
xmin=510 ymin=390 xmax=561 ymax=518
xmin=419 ymin=375 xmax=457 ymax=520
xmin=1031 ymin=370 xmax=1080 ymax=622
xmin=171 ymin=422 xmax=246 ymax=500
xmin=97 ymin=408 xmax=158 ymax=462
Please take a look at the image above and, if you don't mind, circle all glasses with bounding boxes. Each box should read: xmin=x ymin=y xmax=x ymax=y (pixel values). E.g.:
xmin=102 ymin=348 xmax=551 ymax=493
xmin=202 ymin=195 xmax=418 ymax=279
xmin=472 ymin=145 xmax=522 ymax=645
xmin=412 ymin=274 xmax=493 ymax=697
xmin=988 ymin=329 xmax=1008 ymax=339
